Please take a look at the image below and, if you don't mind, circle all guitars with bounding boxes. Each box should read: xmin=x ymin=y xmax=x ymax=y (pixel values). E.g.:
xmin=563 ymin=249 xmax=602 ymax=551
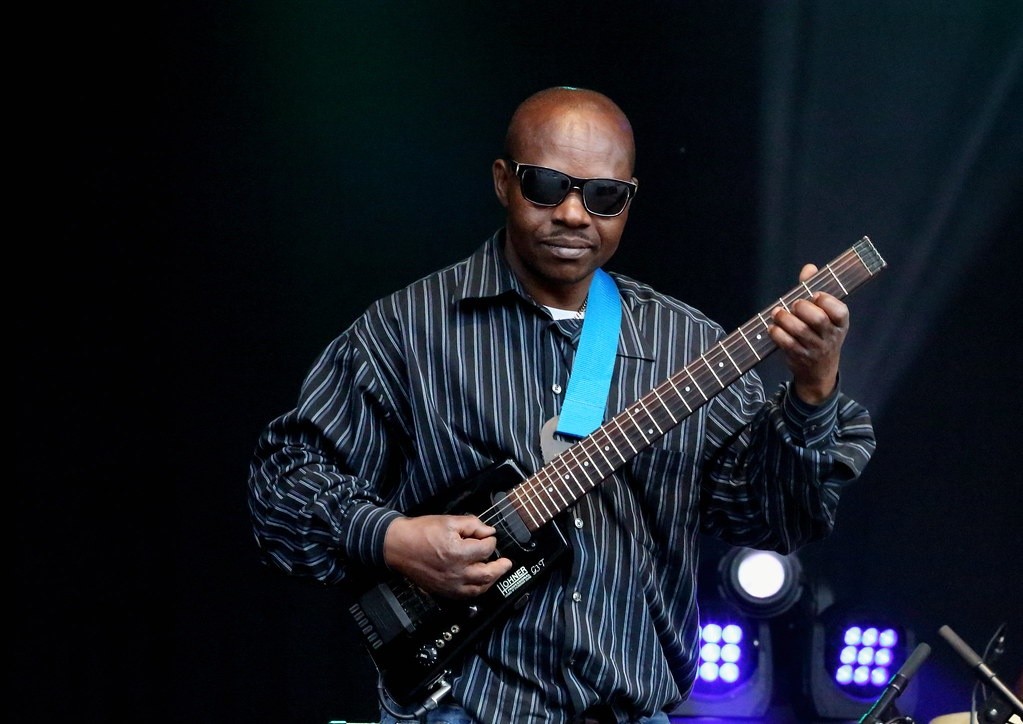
xmin=327 ymin=233 xmax=888 ymax=701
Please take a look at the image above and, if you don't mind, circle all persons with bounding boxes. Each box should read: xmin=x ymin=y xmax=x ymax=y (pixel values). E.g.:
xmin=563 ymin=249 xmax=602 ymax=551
xmin=244 ymin=85 xmax=878 ymax=723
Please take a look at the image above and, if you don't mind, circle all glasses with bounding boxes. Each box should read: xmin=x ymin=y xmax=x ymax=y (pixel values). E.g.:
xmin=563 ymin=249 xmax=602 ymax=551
xmin=505 ymin=158 xmax=640 ymax=218
